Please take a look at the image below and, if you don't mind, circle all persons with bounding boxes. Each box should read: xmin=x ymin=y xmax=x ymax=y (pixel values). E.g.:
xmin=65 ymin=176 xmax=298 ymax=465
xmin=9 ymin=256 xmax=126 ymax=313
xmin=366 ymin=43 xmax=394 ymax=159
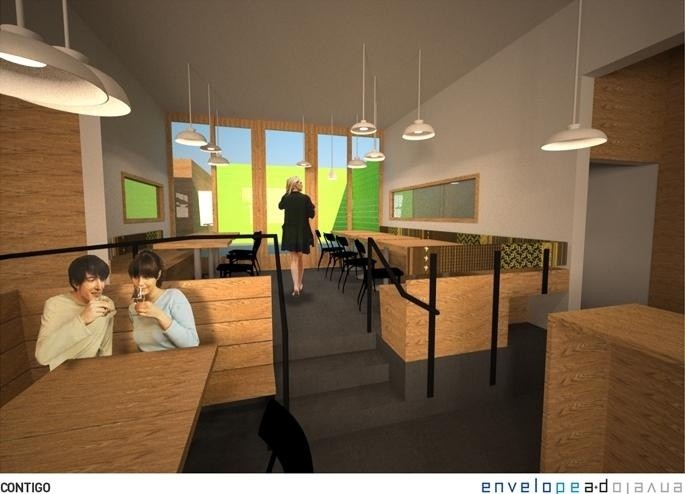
xmin=34 ymin=254 xmax=117 ymax=372
xmin=278 ymin=175 xmax=316 ymax=296
xmin=127 ymin=250 xmax=201 ymax=352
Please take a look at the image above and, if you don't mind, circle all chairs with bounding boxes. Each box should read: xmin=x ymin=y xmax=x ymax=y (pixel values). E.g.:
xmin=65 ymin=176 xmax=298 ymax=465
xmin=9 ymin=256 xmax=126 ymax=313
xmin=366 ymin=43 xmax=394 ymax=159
xmin=0 ymin=218 xmax=683 ymax=472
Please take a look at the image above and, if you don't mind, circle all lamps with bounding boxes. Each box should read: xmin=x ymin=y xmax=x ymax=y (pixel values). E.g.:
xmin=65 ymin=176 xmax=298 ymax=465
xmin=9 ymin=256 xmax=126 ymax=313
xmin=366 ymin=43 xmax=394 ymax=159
xmin=401 ymin=48 xmax=438 ymax=142
xmin=295 ymin=112 xmax=313 ymax=168
xmin=200 ymin=80 xmax=223 ymax=153
xmin=174 ymin=59 xmax=208 ymax=148
xmin=538 ymin=0 xmax=609 ymax=153
xmin=349 ymin=39 xmax=379 ymax=135
xmin=345 ymin=112 xmax=367 ymax=170
xmin=328 ymin=110 xmax=336 ymax=180
xmin=364 ymin=72 xmax=387 ymax=162
xmin=0 ymin=0 xmax=108 ymax=113
xmin=49 ymin=0 xmax=132 ymax=117
xmin=207 ymin=110 xmax=230 ymax=165
